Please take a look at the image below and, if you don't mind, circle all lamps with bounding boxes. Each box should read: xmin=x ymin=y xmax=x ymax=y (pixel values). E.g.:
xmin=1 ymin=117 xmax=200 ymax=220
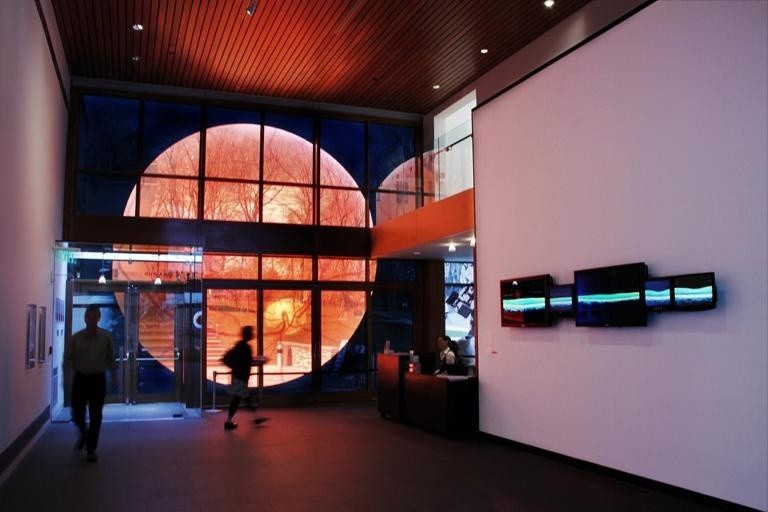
xmin=245 ymin=0 xmax=257 ymax=17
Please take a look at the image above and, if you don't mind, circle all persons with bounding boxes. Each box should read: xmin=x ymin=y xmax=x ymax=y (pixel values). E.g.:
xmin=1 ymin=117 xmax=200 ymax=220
xmin=60 ymin=303 xmax=119 ymax=465
xmin=222 ymin=324 xmax=272 ymax=432
xmin=430 ymin=334 xmax=459 ymax=378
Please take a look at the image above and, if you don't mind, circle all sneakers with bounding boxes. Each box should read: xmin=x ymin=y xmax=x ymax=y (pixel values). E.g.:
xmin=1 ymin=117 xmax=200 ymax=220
xmin=223 ymin=415 xmax=270 ymax=431
xmin=75 ymin=429 xmax=98 ymax=464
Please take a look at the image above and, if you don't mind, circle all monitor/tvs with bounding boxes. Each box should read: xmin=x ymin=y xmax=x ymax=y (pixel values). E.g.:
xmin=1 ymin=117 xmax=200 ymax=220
xmin=644 ymin=271 xmax=717 ymax=313
xmin=574 ymin=261 xmax=647 ymax=329
xmin=548 ymin=284 xmax=575 ymax=315
xmin=500 ymin=273 xmax=555 ymax=330
